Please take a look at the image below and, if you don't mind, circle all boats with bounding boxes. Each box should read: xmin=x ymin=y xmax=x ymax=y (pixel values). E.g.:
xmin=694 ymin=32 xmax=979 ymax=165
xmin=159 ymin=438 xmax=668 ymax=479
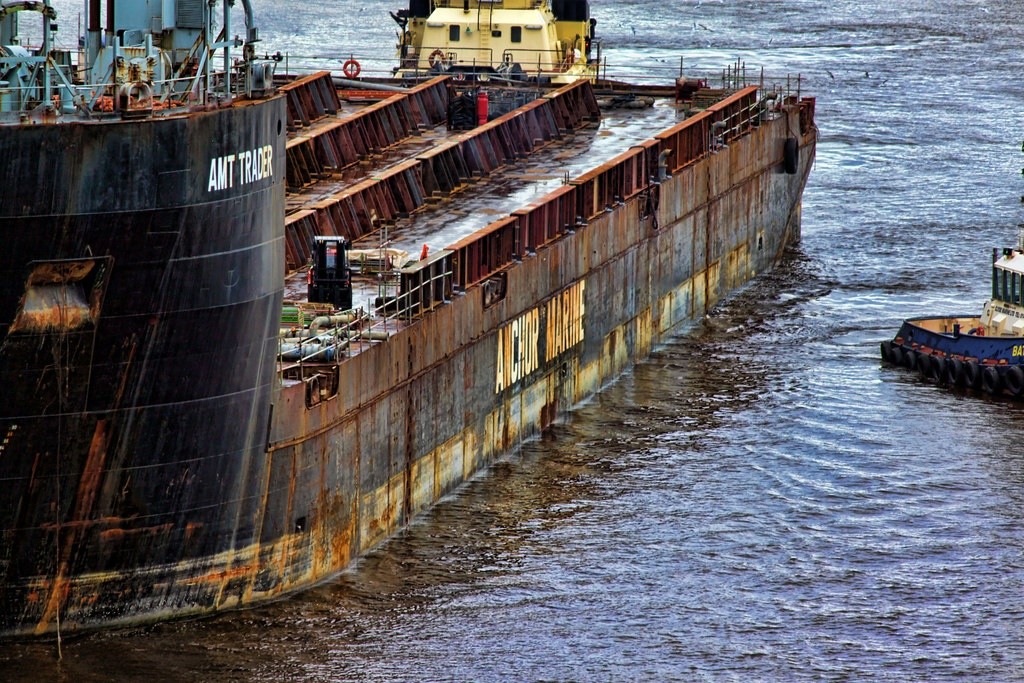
xmin=874 ymin=137 xmax=1024 ymax=403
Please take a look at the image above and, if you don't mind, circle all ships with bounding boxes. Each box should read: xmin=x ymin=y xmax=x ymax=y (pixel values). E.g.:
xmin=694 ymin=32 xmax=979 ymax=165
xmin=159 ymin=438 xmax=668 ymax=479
xmin=1 ymin=0 xmax=825 ymax=633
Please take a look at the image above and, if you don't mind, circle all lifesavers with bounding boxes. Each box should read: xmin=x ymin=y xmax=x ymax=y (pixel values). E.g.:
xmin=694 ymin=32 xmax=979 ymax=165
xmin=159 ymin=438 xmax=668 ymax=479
xmin=343 ymin=59 xmax=361 ymax=78
xmin=429 ymin=50 xmax=445 ymax=69
